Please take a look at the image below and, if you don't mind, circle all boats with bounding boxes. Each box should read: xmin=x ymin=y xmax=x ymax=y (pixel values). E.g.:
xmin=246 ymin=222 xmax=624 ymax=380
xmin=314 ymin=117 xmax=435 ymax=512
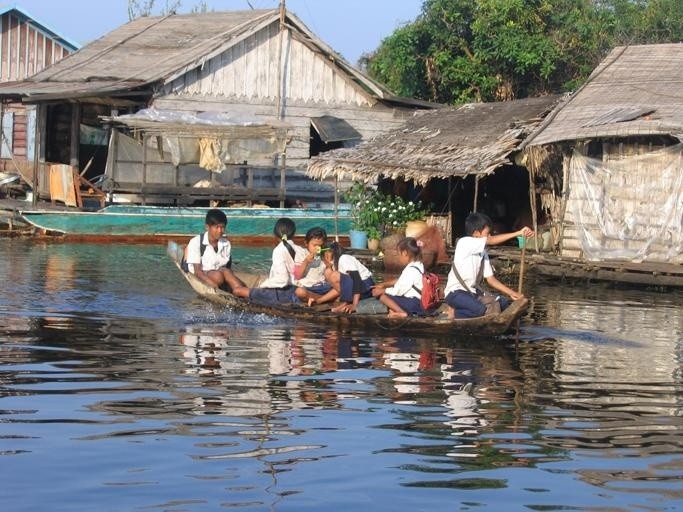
xmin=164 ymin=240 xmax=528 ymax=335
xmin=16 ymin=199 xmax=353 ymax=247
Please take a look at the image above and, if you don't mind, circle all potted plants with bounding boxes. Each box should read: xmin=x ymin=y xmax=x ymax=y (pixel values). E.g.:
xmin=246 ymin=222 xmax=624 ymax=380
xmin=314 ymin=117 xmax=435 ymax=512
xmin=406 ymin=210 xmax=427 ymax=238
xmin=346 ymin=183 xmax=381 ymax=252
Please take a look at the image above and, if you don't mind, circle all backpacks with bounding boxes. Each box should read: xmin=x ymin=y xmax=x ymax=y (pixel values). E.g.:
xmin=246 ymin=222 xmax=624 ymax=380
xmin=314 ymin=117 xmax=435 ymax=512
xmin=181 ymin=232 xmax=206 ymax=273
xmin=411 ymin=265 xmax=440 ymax=310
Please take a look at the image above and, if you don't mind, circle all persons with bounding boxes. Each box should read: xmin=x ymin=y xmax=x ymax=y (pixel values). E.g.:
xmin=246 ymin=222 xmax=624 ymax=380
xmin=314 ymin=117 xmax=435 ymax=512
xmin=371 ymin=237 xmax=436 ymax=318
xmin=444 ymin=213 xmax=535 ymax=320
xmin=186 ymin=209 xmax=247 ymax=291
xmin=232 ymin=218 xmax=374 ymax=315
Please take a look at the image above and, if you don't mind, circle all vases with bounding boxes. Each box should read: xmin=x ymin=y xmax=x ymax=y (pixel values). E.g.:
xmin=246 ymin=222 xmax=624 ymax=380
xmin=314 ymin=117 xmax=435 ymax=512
xmin=384 ymin=223 xmax=407 ymax=242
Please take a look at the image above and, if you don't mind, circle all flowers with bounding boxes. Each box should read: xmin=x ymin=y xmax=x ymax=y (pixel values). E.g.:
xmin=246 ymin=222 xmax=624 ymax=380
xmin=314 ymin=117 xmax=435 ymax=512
xmin=373 ymin=192 xmax=427 ymax=229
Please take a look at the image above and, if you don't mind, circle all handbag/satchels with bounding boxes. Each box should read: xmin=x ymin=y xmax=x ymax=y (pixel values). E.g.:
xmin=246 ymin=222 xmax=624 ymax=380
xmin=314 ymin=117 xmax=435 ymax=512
xmin=479 ymin=296 xmax=501 ymax=316
